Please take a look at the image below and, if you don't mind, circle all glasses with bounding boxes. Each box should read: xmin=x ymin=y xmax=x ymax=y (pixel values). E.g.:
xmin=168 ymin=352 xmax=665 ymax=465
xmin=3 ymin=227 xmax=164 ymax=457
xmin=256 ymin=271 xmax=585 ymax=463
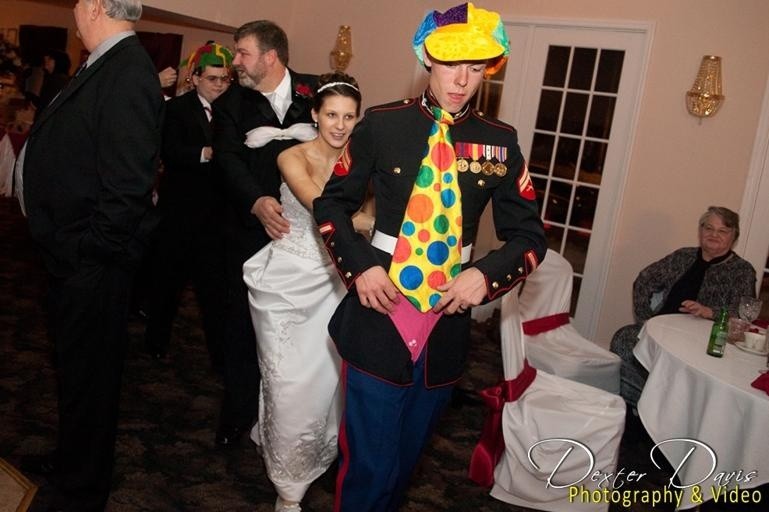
xmin=198 ymin=73 xmax=234 ymax=84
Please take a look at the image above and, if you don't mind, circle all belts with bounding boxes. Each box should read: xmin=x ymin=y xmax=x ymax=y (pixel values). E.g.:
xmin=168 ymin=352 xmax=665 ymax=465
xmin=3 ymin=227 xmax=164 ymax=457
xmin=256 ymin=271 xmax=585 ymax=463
xmin=370 ymin=230 xmax=473 ymax=266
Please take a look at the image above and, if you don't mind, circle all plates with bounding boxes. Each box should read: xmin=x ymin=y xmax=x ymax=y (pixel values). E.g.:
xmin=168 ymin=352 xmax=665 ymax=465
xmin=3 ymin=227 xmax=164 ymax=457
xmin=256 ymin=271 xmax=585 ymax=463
xmin=734 ymin=341 xmax=769 ymax=356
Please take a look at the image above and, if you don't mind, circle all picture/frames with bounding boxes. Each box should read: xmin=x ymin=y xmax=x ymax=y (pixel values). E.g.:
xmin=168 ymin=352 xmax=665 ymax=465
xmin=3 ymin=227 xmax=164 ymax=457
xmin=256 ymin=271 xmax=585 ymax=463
xmin=1 ymin=456 xmax=38 ymax=512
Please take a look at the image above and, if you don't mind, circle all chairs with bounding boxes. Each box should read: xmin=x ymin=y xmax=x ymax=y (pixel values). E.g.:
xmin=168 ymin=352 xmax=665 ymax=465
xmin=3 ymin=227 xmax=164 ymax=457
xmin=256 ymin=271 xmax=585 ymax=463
xmin=467 ymin=281 xmax=626 ymax=512
xmin=515 ymin=248 xmax=621 ymax=394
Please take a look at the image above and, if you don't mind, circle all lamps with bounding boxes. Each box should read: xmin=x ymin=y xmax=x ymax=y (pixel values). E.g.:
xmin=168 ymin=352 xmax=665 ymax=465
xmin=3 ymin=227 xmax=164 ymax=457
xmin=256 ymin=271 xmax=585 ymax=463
xmin=330 ymin=24 xmax=354 ymax=72
xmin=685 ymin=54 xmax=725 ymax=125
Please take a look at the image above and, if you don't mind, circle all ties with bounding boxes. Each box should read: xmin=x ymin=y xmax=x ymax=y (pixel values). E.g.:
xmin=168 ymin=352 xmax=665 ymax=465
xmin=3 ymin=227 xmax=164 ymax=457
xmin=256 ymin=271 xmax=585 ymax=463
xmin=388 ymin=108 xmax=463 ymax=363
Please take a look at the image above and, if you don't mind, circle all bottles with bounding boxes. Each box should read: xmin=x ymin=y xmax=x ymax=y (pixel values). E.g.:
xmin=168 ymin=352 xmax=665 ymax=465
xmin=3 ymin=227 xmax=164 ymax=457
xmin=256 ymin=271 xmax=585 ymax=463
xmin=706 ymin=304 xmax=730 ymax=357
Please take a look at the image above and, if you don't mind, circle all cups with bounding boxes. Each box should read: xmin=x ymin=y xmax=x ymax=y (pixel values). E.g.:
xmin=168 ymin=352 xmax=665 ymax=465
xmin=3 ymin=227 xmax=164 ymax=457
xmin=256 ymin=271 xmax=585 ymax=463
xmin=726 ymin=317 xmax=751 ymax=344
xmin=739 ymin=295 xmax=763 ymax=323
xmin=743 ymin=331 xmax=767 ymax=352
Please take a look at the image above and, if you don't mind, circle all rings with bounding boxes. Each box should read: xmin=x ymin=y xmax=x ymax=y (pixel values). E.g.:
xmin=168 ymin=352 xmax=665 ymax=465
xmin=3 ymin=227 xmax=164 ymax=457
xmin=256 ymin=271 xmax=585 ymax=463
xmin=458 ymin=305 xmax=468 ymax=313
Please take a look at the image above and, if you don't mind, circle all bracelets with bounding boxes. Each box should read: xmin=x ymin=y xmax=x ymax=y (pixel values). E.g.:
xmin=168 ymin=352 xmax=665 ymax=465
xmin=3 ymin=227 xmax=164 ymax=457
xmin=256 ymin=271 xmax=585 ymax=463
xmin=369 ymin=216 xmax=376 ymax=238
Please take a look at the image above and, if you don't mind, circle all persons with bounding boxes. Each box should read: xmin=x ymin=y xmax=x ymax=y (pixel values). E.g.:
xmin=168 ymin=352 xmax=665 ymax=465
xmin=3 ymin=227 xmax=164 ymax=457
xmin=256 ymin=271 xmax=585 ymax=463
xmin=158 ymin=63 xmax=178 ymax=87
xmin=159 ymin=38 xmax=231 ymax=188
xmin=192 ymin=20 xmax=320 ymax=454
xmin=609 ymin=206 xmax=759 ymax=412
xmin=310 ymin=0 xmax=550 ymax=511
xmin=13 ymin=0 xmax=163 ymax=511
xmin=239 ymin=68 xmax=378 ymax=511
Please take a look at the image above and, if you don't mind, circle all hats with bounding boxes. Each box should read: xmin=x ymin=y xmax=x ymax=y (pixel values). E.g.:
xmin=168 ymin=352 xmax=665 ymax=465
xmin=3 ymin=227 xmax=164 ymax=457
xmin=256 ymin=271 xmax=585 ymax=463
xmin=179 ymin=40 xmax=233 ymax=68
xmin=412 ymin=3 xmax=511 ymax=79
